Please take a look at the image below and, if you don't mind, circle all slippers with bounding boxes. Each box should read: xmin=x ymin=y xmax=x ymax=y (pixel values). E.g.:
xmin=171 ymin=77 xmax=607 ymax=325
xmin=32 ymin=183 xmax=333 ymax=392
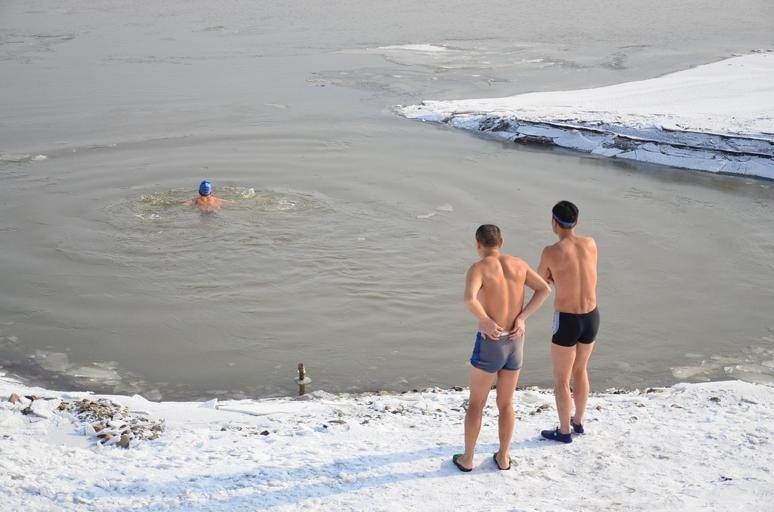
xmin=453 ymin=455 xmax=472 ymax=472
xmin=494 ymin=452 xmax=511 ymax=469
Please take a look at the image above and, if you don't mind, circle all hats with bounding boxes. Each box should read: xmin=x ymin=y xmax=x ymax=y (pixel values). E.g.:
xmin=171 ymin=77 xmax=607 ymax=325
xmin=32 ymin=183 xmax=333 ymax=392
xmin=200 ymin=181 xmax=211 ymax=194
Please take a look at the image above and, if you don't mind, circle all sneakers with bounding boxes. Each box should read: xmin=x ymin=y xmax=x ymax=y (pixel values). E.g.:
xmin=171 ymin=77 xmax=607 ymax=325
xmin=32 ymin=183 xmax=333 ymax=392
xmin=541 ymin=416 xmax=583 ymax=443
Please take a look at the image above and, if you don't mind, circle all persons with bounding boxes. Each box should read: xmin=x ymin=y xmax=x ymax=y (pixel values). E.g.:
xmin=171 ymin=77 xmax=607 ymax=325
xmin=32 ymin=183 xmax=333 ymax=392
xmin=451 ymin=223 xmax=551 ymax=471
xmin=537 ymin=200 xmax=601 ymax=444
xmin=182 ymin=179 xmax=235 ymax=213
